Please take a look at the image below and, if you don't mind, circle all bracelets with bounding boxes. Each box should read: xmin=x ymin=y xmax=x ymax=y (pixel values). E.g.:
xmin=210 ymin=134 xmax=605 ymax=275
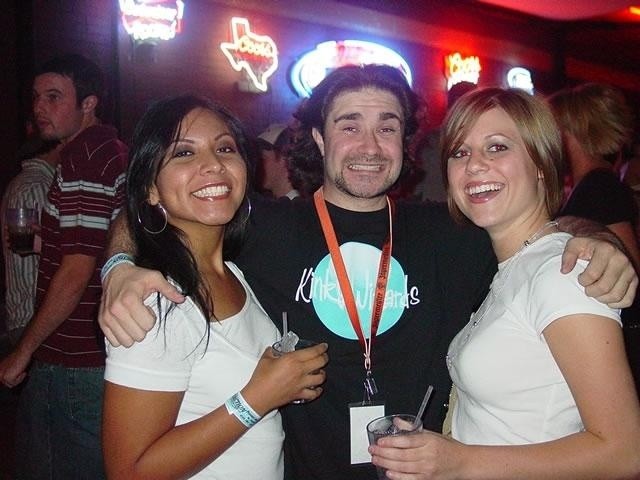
xmin=224 ymin=392 xmax=263 ymax=429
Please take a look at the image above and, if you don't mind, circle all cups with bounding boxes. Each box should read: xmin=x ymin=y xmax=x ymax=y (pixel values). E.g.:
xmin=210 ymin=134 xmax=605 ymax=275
xmin=6 ymin=208 xmax=37 ymax=250
xmin=271 ymin=340 xmax=319 ymax=405
xmin=366 ymin=413 xmax=423 ymax=480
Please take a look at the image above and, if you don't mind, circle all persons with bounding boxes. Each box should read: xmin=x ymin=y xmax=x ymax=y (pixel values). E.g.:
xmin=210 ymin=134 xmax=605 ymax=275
xmin=0 ymin=56 xmax=129 ymax=479
xmin=98 ymin=61 xmax=639 ymax=479
xmin=103 ymin=90 xmax=328 ymax=480
xmin=254 ymin=80 xmax=639 ymax=397
xmin=367 ymin=85 xmax=640 ymax=479
